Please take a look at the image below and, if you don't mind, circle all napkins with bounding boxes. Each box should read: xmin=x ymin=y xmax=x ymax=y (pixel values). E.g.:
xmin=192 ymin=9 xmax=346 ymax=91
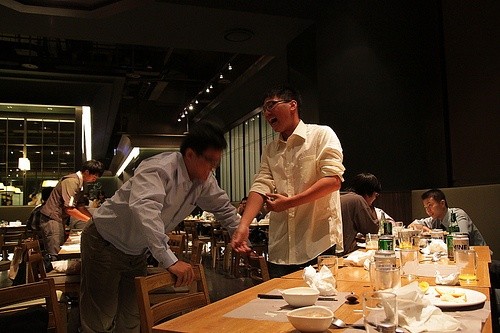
xmin=302 ymin=263 xmax=338 ymax=296
xmin=345 ymin=248 xmax=376 ymax=270
xmin=377 ymin=282 xmax=462 ymax=332
xmin=421 ymin=240 xmax=448 ymax=257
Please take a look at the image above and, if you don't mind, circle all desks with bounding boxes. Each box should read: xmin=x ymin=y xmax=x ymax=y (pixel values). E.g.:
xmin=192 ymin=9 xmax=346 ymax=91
xmin=58 ymin=230 xmax=81 ymax=258
xmin=155 ymin=277 xmax=494 ymax=333
xmin=281 ymin=260 xmax=491 ymax=287
xmin=342 ymin=245 xmax=491 ymax=263
xmin=0 ymin=223 xmax=26 ymax=259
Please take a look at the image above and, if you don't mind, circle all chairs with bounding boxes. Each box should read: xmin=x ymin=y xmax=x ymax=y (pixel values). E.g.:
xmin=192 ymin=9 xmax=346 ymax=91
xmin=0 ymin=233 xmax=68 ymax=333
xmin=169 ymin=221 xmax=271 ymax=283
xmin=135 ymin=263 xmax=211 ymax=333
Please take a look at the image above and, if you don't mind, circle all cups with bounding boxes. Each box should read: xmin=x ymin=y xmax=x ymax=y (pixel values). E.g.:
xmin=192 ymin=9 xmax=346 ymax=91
xmin=400 ymin=250 xmax=418 ymax=277
xmin=363 ymin=292 xmax=398 ymax=333
xmin=456 ymin=251 xmax=476 ymax=285
xmin=375 ymin=251 xmax=395 ymax=256
xmin=414 ymin=224 xmax=424 ymax=244
xmin=369 ymin=262 xmax=392 ymax=291
xmin=375 ymin=267 xmax=400 ymax=290
xmin=374 ymin=255 xmax=396 ymax=271
xmin=366 ymin=234 xmax=378 ymax=251
xmin=318 ymin=255 xmax=338 ymax=289
xmin=399 ymin=232 xmax=412 ymax=250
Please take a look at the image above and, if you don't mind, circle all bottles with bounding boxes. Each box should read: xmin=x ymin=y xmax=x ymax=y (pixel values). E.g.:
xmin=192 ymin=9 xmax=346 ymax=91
xmin=379 ymin=213 xmax=386 ymax=234
xmin=448 ymin=213 xmax=460 ymax=261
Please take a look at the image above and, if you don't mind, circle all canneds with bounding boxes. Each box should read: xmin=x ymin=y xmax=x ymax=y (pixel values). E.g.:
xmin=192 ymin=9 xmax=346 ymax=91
xmin=447 ymin=235 xmax=469 ymax=264
xmin=374 ymin=251 xmax=396 ymax=289
xmin=431 ymin=231 xmax=444 ymax=242
xmin=383 ymin=221 xmax=392 ymax=234
xmin=377 ymin=237 xmax=393 ymax=250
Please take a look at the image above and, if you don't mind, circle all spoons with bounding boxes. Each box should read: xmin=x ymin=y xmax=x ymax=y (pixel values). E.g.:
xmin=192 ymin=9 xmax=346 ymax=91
xmin=331 ymin=316 xmax=364 ymax=327
xmin=345 ymin=292 xmax=359 ymax=300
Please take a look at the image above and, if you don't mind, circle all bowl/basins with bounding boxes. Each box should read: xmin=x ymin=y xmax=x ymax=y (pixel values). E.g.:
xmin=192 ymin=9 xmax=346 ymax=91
xmin=282 ymin=286 xmax=320 ymax=307
xmin=287 ymin=306 xmax=334 ymax=333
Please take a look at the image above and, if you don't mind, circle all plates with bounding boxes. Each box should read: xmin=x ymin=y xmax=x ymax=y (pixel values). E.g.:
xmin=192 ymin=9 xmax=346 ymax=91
xmin=429 ymin=286 xmax=486 ymax=306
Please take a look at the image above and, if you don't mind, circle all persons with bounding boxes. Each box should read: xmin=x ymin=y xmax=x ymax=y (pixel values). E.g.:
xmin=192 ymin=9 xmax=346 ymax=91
xmin=237 ymin=197 xmax=270 ymax=222
xmin=40 ymin=160 xmax=104 ymax=272
xmin=76 ymin=190 xmax=109 ymax=218
xmin=334 ymin=173 xmax=381 ymax=257
xmin=6 ymin=199 xmax=11 ymax=205
xmin=407 ymin=190 xmax=496 ymax=289
xmin=80 ymin=123 xmax=254 ymax=333
xmin=229 ymin=86 xmax=345 ymax=280
xmin=28 ymin=194 xmax=44 ymax=206
xmin=346 ymin=186 xmax=396 ymax=227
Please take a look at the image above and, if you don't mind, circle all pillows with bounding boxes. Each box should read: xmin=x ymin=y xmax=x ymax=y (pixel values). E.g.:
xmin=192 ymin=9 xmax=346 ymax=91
xmin=49 ymin=258 xmax=82 ymax=274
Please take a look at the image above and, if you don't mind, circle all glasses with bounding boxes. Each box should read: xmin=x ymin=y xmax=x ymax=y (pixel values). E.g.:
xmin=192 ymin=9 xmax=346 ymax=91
xmin=202 ymin=152 xmax=221 ymax=166
xmin=261 ymin=99 xmax=289 ymax=113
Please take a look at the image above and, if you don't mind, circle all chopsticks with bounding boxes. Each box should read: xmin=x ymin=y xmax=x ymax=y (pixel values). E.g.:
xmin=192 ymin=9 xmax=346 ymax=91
xmin=258 ymin=294 xmax=335 ymax=300
